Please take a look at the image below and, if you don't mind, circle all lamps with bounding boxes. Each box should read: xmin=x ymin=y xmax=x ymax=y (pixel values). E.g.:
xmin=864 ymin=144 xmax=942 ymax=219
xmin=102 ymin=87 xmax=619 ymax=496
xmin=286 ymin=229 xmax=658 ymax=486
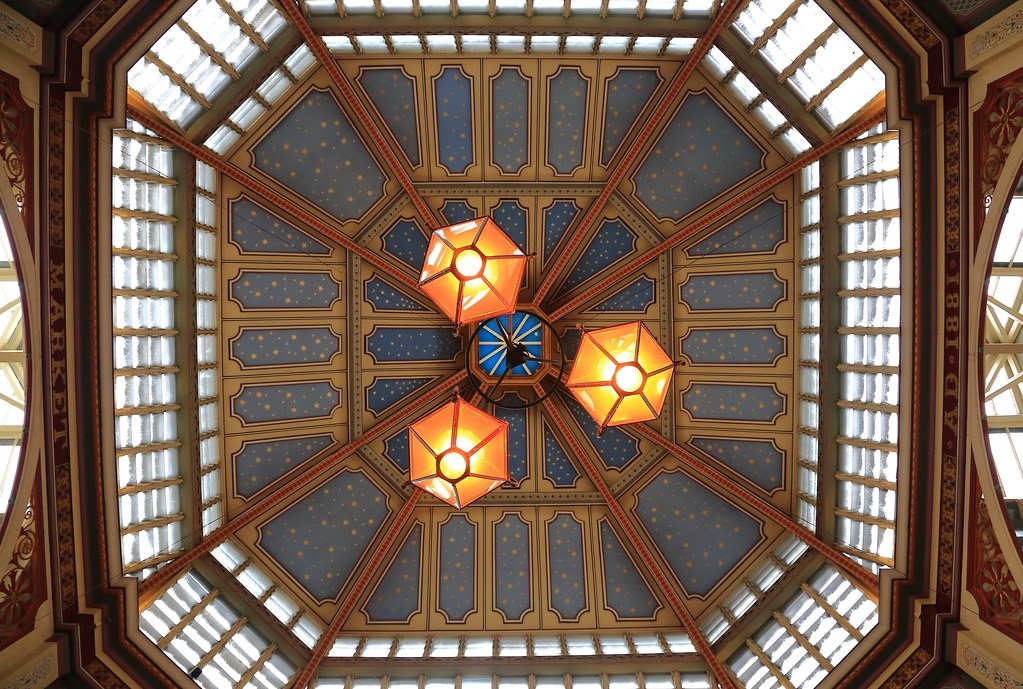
xmin=409 ymin=213 xmax=686 ymax=508
